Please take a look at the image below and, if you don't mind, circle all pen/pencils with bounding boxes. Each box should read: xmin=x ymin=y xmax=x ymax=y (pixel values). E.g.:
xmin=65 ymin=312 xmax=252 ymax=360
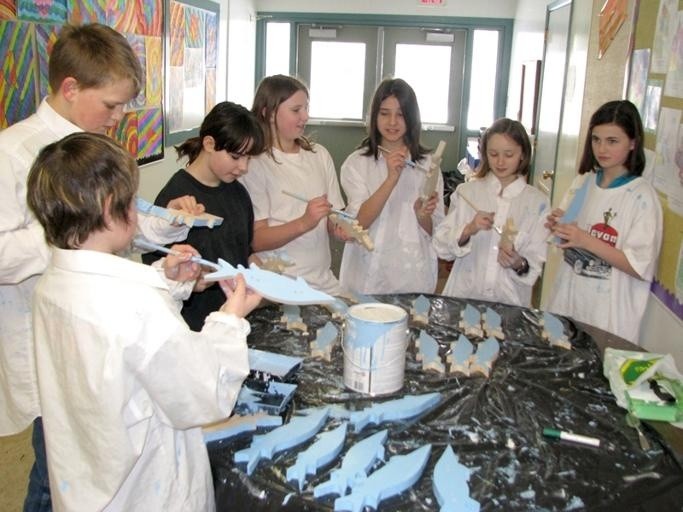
xmin=542 ymin=428 xmax=600 ymax=447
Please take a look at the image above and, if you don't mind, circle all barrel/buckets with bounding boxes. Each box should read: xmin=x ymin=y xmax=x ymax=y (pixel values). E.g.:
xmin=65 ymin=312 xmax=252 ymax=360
xmin=335 ymin=302 xmax=413 ymax=397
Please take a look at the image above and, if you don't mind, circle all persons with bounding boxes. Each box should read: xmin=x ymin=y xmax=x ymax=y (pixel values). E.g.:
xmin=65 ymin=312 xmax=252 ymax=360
xmin=540 ymin=98 xmax=667 ymax=351
xmin=336 ymin=74 xmax=447 ymax=298
xmin=23 ymin=130 xmax=264 ymax=512
xmin=433 ymin=114 xmax=557 ymax=311
xmin=236 ymin=73 xmax=355 ymax=302
xmin=0 ymin=20 xmax=207 ymax=512
xmin=138 ymin=97 xmax=269 ymax=333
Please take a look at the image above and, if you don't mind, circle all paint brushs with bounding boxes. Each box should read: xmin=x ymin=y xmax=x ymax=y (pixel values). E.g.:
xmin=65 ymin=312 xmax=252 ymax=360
xmin=131 ymin=238 xmax=219 ymax=270
xmin=377 ymin=145 xmax=417 ymax=167
xmin=281 ymin=190 xmax=355 ymax=218
xmin=457 ymin=191 xmax=503 ymax=235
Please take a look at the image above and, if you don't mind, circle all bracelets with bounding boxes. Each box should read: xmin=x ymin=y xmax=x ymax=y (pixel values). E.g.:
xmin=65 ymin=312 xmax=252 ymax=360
xmin=515 ymin=259 xmax=528 ymax=272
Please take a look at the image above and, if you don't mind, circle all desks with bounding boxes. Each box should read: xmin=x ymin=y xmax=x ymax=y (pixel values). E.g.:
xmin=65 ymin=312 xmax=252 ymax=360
xmin=200 ymin=292 xmax=682 ymax=512
